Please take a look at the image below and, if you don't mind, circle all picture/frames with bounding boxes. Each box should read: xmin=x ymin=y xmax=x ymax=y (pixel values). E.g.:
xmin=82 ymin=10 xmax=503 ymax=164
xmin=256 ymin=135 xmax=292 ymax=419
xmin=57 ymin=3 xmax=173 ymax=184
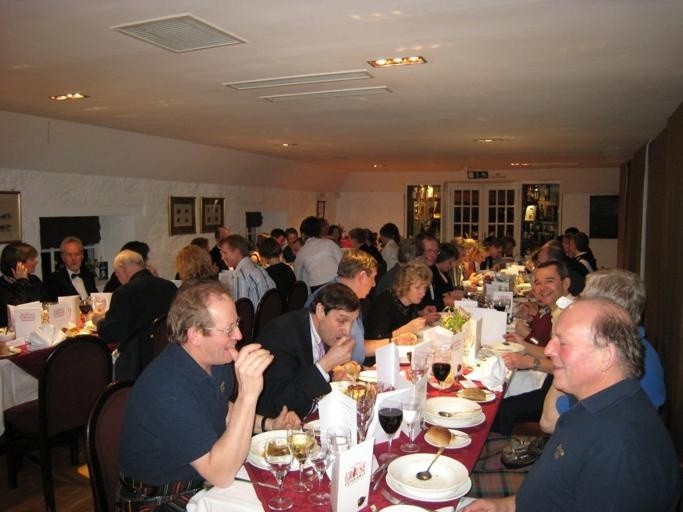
xmin=0 ymin=190 xmax=23 ymax=245
xmin=169 ymin=196 xmax=196 ymax=234
xmin=201 ymin=196 xmax=224 ymax=234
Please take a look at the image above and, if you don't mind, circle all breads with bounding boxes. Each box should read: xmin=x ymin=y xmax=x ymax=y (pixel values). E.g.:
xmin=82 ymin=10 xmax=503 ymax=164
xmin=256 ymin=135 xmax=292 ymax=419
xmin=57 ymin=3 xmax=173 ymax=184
xmin=462 ymin=388 xmax=487 ymax=401
xmin=427 ymin=426 xmax=452 ymax=446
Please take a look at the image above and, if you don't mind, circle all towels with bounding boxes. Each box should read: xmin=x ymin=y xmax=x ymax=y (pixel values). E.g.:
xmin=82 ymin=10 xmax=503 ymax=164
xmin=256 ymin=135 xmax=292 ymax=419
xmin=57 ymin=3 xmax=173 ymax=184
xmin=467 ymin=354 xmax=507 ymax=391
xmin=32 ymin=325 xmax=66 ymax=349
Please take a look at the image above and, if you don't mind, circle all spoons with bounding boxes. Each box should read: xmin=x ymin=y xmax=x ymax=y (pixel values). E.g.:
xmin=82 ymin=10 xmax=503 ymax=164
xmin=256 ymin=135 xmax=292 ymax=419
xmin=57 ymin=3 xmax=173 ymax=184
xmin=438 ymin=407 xmax=481 ymax=417
xmin=415 ymin=446 xmax=444 ymax=481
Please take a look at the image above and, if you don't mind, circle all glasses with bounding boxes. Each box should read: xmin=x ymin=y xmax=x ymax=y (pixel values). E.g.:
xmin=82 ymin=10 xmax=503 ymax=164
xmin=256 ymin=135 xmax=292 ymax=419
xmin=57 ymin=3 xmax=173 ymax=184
xmin=426 ymin=248 xmax=440 ymax=256
xmin=203 ymin=318 xmax=242 ymax=335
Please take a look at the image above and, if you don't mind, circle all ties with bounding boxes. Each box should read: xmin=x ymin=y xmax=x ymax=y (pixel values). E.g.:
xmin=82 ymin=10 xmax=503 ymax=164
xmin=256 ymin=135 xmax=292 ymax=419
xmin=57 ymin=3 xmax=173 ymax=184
xmin=318 ymin=341 xmax=326 ymax=359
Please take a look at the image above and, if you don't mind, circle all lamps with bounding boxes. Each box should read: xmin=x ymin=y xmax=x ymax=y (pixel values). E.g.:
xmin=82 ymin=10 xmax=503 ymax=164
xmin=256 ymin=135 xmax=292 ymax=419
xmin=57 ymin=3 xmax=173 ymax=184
xmin=368 ymin=55 xmax=427 ymax=69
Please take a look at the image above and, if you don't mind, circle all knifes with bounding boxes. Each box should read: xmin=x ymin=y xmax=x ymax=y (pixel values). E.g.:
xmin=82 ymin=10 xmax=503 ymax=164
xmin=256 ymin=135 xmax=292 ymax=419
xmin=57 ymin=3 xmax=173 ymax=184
xmin=234 ymin=477 xmax=280 ymax=490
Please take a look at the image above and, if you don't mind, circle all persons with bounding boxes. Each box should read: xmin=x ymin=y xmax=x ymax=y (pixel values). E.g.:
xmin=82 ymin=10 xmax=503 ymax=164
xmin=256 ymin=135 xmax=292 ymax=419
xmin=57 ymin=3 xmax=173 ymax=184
xmin=221 ymin=234 xmax=276 ymax=314
xmin=454 ymin=298 xmax=682 ymax=511
xmin=103 ymin=241 xmax=158 ymax=292
xmin=250 ymin=232 xmax=271 ymax=266
xmin=259 ymin=238 xmax=297 ymax=290
xmin=537 ymin=227 xmax=596 ymax=246
xmin=503 ymin=261 xmax=578 ymax=376
xmin=419 ymin=232 xmax=461 ymax=309
xmin=448 ymin=237 xmax=483 ymax=293
xmin=328 ymin=225 xmax=343 ymax=248
xmin=175 ymin=237 xmax=211 ymax=281
xmin=374 ymin=238 xmax=426 ymax=312
xmin=319 ymin=218 xmax=337 ymax=240
xmin=293 ymin=216 xmax=343 ymax=294
xmin=432 ymin=244 xmax=466 ymax=296
xmin=380 ymin=226 xmax=399 ymax=272
xmin=176 ymin=244 xmax=218 ymax=290
xmin=539 ymin=269 xmax=665 ymax=435
xmin=210 ymin=226 xmax=231 ymax=273
xmin=233 ymin=283 xmax=361 ymax=422
xmin=92 ymin=249 xmax=178 ymax=382
xmin=524 ymin=247 xmax=584 ymax=318
xmin=464 ymin=239 xmax=495 ymax=281
xmin=364 ymin=228 xmax=387 ymax=278
xmin=0 ymin=241 xmax=55 ymax=327
xmin=517 ymin=268 xmax=552 ymax=347
xmin=348 ymin=228 xmax=376 ymax=258
xmin=304 ymin=249 xmax=417 ymax=365
xmin=271 ymin=228 xmax=287 ymax=262
xmin=292 ymin=238 xmax=304 ymax=257
xmin=475 ymin=237 xmax=515 ymax=272
xmin=49 ymin=237 xmax=99 ymax=300
xmin=116 ymin=279 xmax=301 ymax=512
xmin=283 ymin=227 xmax=298 ymax=262
xmin=364 ymin=261 xmax=433 ymax=341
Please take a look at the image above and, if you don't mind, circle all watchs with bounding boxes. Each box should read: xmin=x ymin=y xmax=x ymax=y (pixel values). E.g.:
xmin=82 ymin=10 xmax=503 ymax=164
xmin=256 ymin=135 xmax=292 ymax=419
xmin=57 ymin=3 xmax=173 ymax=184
xmin=533 ymin=358 xmax=540 ymax=369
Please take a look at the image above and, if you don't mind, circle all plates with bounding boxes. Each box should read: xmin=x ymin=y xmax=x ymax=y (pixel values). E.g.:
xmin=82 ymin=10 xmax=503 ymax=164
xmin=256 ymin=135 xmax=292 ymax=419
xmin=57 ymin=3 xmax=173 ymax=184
xmin=246 ymin=429 xmax=321 ymax=472
xmin=387 ymin=453 xmax=468 ymax=498
xmin=423 ymin=428 xmax=472 ymax=449
xmin=383 ymin=473 xmax=473 ymax=503
xmin=488 ymin=341 xmax=525 ymax=355
xmin=425 ymin=396 xmax=483 ymax=424
xmin=456 ymin=388 xmax=496 ymax=404
xmin=423 ymin=413 xmax=488 ymax=429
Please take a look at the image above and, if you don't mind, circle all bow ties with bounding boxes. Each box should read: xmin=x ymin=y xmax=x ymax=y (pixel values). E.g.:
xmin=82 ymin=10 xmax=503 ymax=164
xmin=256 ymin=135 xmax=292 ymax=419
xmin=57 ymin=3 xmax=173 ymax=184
xmin=71 ymin=273 xmax=82 ymax=279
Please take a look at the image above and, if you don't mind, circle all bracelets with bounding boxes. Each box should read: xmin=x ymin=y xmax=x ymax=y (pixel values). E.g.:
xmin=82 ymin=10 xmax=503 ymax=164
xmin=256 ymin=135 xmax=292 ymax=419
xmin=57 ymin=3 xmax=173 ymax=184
xmin=524 ymin=334 xmax=539 ymax=345
xmin=262 ymin=416 xmax=267 ymax=433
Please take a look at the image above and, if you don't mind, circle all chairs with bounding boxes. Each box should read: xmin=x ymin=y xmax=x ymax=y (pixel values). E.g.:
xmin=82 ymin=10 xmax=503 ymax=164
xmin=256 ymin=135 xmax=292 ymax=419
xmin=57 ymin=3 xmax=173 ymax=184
xmin=86 ymin=381 xmax=135 ymax=512
xmin=6 ymin=335 xmax=113 ymax=512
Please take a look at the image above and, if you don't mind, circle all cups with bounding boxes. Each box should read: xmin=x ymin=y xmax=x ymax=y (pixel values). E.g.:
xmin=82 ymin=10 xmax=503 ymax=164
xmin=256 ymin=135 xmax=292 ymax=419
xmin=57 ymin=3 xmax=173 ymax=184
xmin=326 ymin=425 xmax=351 ymax=487
xmin=379 ymin=401 xmax=403 ymax=462
xmin=307 ymin=439 xmax=335 ymax=506
xmin=351 ymin=394 xmax=374 ymax=443
xmin=399 ymin=397 xmax=422 ymax=453
xmin=493 ymin=298 xmax=506 ymax=311
xmin=432 ymin=346 xmax=452 ymax=397
xmin=264 ymin=439 xmax=296 ymax=512
xmin=78 ymin=300 xmax=91 ymax=315
xmin=94 ymin=297 xmax=107 ymax=312
xmin=286 ymin=425 xmax=314 ymax=494
